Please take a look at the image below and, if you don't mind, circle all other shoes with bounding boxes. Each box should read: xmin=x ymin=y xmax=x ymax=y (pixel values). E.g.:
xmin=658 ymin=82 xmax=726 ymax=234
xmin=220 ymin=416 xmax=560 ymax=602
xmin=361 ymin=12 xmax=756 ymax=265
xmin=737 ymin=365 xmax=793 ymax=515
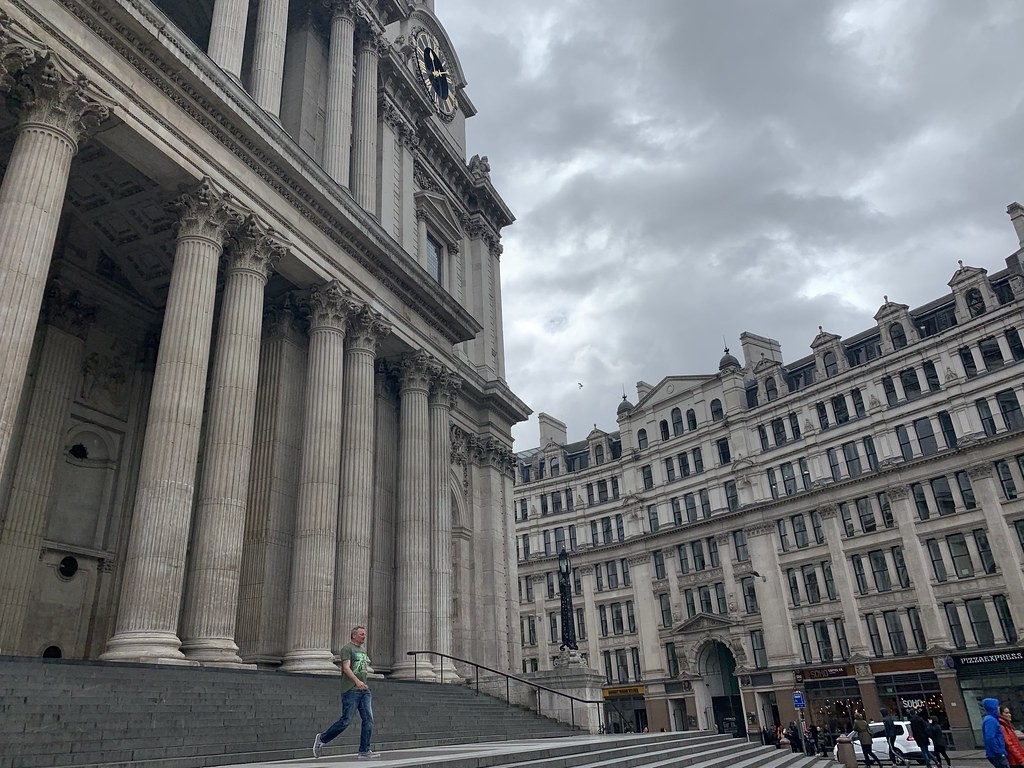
xmin=937 ymin=762 xmax=942 ymax=768
xmin=891 ymin=766 xmax=897 ymax=768
xmin=904 ymin=759 xmax=910 ymax=768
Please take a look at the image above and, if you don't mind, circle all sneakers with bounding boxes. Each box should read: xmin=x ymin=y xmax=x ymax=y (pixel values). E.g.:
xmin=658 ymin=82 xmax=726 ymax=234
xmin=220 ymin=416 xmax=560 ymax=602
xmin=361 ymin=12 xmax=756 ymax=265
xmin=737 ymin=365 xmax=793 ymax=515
xmin=313 ymin=733 xmax=325 ymax=758
xmin=358 ymin=750 xmax=381 ymax=759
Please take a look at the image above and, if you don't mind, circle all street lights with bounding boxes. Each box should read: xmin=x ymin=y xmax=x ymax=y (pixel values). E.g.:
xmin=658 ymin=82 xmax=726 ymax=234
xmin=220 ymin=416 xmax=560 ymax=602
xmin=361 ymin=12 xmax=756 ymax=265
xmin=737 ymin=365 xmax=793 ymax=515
xmin=558 ymin=544 xmax=579 ymax=650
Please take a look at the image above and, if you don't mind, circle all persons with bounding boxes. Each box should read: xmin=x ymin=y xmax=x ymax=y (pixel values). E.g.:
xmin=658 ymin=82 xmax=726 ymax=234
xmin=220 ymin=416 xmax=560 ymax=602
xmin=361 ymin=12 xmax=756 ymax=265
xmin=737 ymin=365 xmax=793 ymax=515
xmin=313 ymin=626 xmax=380 ymax=759
xmin=661 ymin=728 xmax=666 ymax=732
xmin=909 ymin=709 xmax=942 ymax=768
xmin=879 ymin=708 xmax=910 ymax=768
xmin=624 ymin=721 xmax=636 ymax=733
xmin=999 ymin=706 xmax=1024 ymax=768
xmin=642 ymin=725 xmax=649 ymax=733
xmin=981 ymin=698 xmax=1010 ymax=768
xmin=853 ymin=713 xmax=883 ymax=768
xmin=927 ymin=716 xmax=953 ymax=768
xmin=763 ymin=721 xmax=828 ymax=757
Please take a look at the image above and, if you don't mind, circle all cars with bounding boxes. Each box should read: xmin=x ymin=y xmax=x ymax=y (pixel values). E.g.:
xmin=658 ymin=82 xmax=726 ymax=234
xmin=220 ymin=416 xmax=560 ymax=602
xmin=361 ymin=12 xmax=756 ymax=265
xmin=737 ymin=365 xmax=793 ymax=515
xmin=833 ymin=720 xmax=935 ymax=766
